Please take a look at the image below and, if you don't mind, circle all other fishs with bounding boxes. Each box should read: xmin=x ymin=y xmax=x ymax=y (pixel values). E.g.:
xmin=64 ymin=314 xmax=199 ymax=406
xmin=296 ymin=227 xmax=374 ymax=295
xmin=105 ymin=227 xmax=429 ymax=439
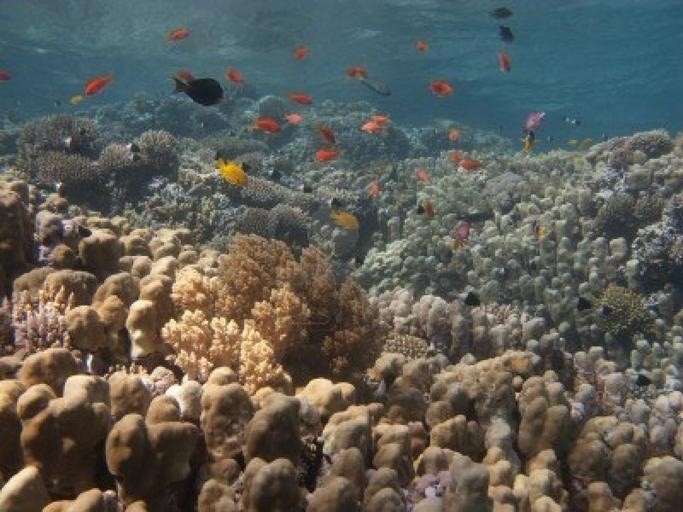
xmin=162 ymin=26 xmax=593 ymax=244
